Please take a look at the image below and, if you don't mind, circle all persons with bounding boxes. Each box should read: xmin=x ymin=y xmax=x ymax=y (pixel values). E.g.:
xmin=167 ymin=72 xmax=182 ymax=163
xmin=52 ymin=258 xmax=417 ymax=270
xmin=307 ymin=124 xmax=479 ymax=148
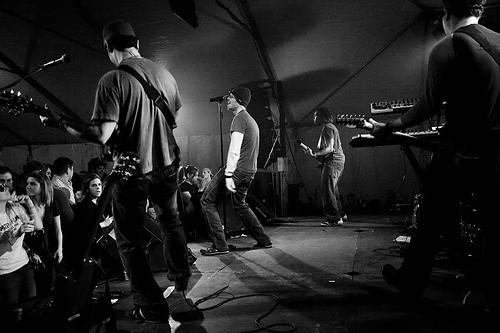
xmin=0 ymin=157 xmax=212 ymax=333
xmin=301 ymin=107 xmax=347 ymax=227
xmin=199 ymin=86 xmax=273 ymax=256
xmin=39 ymin=19 xmax=191 ymax=323
xmin=369 ymin=0 xmax=500 ymax=296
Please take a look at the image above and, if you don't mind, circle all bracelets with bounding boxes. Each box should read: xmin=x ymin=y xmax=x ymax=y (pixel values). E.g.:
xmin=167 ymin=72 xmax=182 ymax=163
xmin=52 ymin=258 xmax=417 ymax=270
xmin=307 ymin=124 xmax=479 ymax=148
xmin=225 ymin=175 xmax=232 ymax=178
xmin=385 ymin=120 xmax=397 ymax=134
xmin=314 ymin=151 xmax=317 ymax=158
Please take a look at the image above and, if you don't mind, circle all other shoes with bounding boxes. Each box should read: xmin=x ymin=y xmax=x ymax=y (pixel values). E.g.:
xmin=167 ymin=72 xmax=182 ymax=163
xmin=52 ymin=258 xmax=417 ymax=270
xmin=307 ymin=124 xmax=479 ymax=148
xmin=341 ymin=214 xmax=347 ymax=220
xmin=321 ymin=218 xmax=344 ymax=226
xmin=381 ymin=263 xmax=410 ymax=294
xmin=175 ymin=281 xmax=188 ymax=292
xmin=199 ymin=247 xmax=229 ymax=254
xmin=253 ymin=243 xmax=273 ymax=248
xmin=131 ymin=307 xmax=158 ymax=322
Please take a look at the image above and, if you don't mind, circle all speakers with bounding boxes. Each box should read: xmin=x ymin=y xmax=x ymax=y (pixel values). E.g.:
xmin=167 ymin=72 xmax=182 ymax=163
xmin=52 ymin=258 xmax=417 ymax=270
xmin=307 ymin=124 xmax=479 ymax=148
xmin=238 ymin=81 xmax=279 ymax=170
xmin=195 ymin=195 xmax=248 ymax=236
xmin=95 ymin=211 xmax=197 ymax=273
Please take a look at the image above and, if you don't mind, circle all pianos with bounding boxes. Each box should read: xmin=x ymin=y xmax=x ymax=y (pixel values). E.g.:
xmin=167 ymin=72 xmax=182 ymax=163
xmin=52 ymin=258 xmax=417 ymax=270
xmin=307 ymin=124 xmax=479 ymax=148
xmin=348 ymin=129 xmax=437 ymax=148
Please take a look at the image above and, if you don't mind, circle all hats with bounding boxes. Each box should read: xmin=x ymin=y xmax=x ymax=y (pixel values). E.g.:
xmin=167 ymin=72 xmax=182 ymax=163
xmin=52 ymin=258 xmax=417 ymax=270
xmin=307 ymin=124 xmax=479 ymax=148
xmin=230 ymin=88 xmax=251 ymax=108
xmin=202 ymin=168 xmax=214 ymax=177
xmin=104 ymin=19 xmax=135 ymax=48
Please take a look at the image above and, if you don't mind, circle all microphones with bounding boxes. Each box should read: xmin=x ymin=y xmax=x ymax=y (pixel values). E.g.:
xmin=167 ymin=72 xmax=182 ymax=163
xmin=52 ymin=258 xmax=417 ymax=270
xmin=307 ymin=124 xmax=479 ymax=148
xmin=40 ymin=54 xmax=70 ymax=69
xmin=209 ymin=96 xmax=228 ymax=102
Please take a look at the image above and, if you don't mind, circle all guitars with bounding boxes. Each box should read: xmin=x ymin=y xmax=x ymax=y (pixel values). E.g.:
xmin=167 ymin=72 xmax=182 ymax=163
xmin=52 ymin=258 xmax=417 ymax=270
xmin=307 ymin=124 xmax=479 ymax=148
xmin=66 ymin=154 xmax=135 ymax=325
xmin=0 ymin=89 xmax=120 ymax=162
xmin=335 ymin=113 xmax=438 ymax=154
xmin=296 ymin=137 xmax=324 ymax=168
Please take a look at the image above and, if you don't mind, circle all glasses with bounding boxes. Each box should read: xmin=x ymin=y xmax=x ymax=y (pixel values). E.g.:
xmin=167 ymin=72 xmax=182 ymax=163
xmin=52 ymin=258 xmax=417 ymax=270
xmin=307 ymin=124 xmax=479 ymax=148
xmin=0 ymin=184 xmax=7 ymax=192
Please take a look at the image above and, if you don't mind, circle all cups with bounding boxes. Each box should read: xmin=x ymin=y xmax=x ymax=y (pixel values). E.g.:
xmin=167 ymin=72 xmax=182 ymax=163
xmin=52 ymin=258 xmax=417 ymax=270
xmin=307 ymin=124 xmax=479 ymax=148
xmin=23 ymin=214 xmax=35 ymax=233
